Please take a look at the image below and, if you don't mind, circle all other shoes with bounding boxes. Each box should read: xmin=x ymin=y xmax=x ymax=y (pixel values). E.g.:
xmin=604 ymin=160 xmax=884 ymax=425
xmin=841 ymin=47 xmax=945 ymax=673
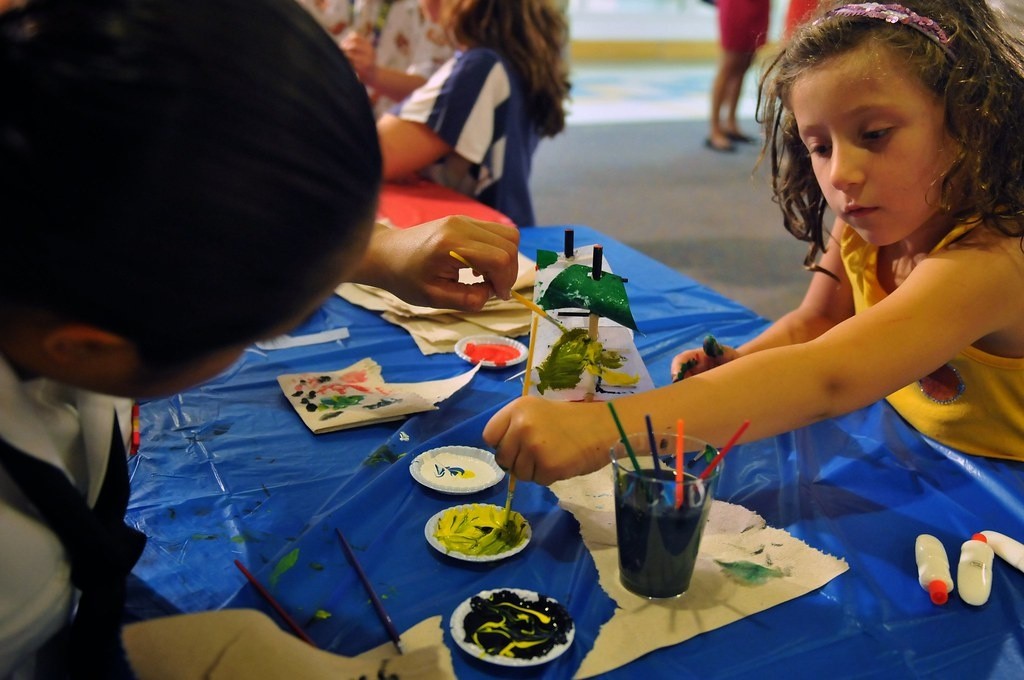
xmin=705 ymin=139 xmax=734 ymax=152
xmin=725 ymin=133 xmax=749 ymax=142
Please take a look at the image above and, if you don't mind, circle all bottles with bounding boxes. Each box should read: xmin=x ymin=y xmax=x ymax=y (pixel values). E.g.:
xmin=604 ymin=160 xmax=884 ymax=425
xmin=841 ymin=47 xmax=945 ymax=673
xmin=981 ymin=530 xmax=1024 ymax=573
xmin=956 ymin=533 xmax=994 ymax=606
xmin=915 ymin=534 xmax=954 ymax=606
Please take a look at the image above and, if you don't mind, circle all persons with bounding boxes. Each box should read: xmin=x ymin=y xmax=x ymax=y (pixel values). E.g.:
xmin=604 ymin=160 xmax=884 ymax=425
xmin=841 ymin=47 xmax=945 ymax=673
xmin=296 ymin=1 xmax=573 ymax=230
xmin=705 ymin=2 xmax=771 ymax=152
xmin=0 ymin=1 xmax=519 ymax=680
xmin=482 ymin=0 xmax=1024 ymax=487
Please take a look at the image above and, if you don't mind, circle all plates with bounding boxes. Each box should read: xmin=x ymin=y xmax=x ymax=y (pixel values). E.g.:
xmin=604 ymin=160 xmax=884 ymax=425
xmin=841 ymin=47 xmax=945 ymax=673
xmin=454 ymin=334 xmax=529 ymax=369
xmin=409 ymin=444 xmax=506 ymax=494
xmin=449 ymin=588 xmax=575 ymax=667
xmin=423 ymin=503 xmax=532 ymax=563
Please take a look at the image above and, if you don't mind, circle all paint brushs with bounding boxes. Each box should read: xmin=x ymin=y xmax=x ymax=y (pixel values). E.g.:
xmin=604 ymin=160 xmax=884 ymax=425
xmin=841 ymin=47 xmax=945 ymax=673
xmin=500 ymin=315 xmax=536 ymax=524
xmin=449 ymin=250 xmax=571 ymax=338
xmin=607 ymin=401 xmax=750 ymax=516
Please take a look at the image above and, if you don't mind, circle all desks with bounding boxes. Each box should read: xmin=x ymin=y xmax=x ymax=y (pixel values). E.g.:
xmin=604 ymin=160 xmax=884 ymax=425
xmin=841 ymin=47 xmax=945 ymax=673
xmin=112 ymin=223 xmax=1022 ymax=680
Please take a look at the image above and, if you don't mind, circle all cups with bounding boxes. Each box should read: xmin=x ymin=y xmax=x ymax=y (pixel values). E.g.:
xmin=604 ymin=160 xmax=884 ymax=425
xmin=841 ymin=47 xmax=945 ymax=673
xmin=608 ymin=431 xmax=725 ymax=602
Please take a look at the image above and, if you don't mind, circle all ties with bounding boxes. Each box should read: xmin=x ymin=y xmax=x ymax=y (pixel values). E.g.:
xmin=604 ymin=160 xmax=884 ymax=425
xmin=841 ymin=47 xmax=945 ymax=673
xmin=1 ymin=405 xmax=149 ymax=680
